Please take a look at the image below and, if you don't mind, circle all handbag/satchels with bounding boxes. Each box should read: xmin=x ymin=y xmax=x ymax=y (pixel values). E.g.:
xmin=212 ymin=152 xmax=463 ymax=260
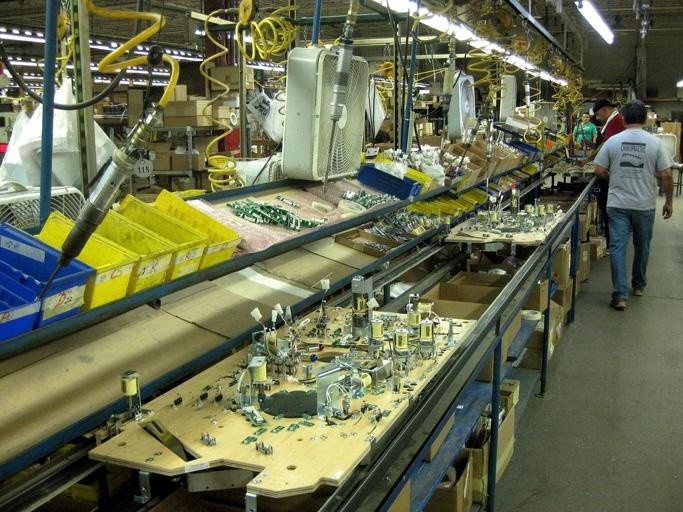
xmin=580 ymin=124 xmax=591 ymax=148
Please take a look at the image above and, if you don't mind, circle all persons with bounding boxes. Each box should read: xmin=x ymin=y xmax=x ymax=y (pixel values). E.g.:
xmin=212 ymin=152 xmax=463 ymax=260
xmin=592 ymin=99 xmax=675 ymax=310
xmin=580 ymin=99 xmax=626 ymax=166
xmin=573 ymin=111 xmax=599 ymax=148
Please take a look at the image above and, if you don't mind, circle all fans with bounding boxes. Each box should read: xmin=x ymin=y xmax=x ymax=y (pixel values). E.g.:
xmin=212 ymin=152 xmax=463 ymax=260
xmin=448 ymin=72 xmax=478 ymax=142
xmin=498 ymin=74 xmax=517 ymax=122
xmin=280 ymin=45 xmax=367 ymax=181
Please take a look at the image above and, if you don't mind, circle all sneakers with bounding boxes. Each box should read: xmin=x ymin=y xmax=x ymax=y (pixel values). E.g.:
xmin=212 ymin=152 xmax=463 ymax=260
xmin=631 ymin=288 xmax=644 ymax=296
xmin=611 ymin=299 xmax=627 ymax=309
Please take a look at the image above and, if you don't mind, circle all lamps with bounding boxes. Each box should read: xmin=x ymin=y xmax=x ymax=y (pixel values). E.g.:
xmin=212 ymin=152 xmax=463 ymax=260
xmin=573 ymin=0 xmax=616 ymax=46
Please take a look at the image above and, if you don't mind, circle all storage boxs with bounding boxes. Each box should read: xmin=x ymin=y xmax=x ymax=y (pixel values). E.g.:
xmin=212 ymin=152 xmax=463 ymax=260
xmin=374 ymin=154 xmax=430 ymax=192
xmin=116 ymin=62 xmax=258 ymax=191
xmin=1 ymin=188 xmax=240 ymax=348
xmin=363 ymin=154 xmax=422 ymax=203
xmin=425 ymin=451 xmax=471 ymax=512
xmin=416 ymin=113 xmax=611 ymax=370
xmin=470 ymin=381 xmax=515 ymax=500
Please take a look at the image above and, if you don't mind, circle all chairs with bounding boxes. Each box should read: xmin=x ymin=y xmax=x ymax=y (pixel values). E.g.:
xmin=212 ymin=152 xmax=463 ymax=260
xmin=656 ymin=133 xmax=683 ymax=197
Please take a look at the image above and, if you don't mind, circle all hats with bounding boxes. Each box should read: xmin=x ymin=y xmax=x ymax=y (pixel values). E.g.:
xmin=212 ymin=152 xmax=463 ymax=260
xmin=593 ymin=98 xmax=620 ymax=113
